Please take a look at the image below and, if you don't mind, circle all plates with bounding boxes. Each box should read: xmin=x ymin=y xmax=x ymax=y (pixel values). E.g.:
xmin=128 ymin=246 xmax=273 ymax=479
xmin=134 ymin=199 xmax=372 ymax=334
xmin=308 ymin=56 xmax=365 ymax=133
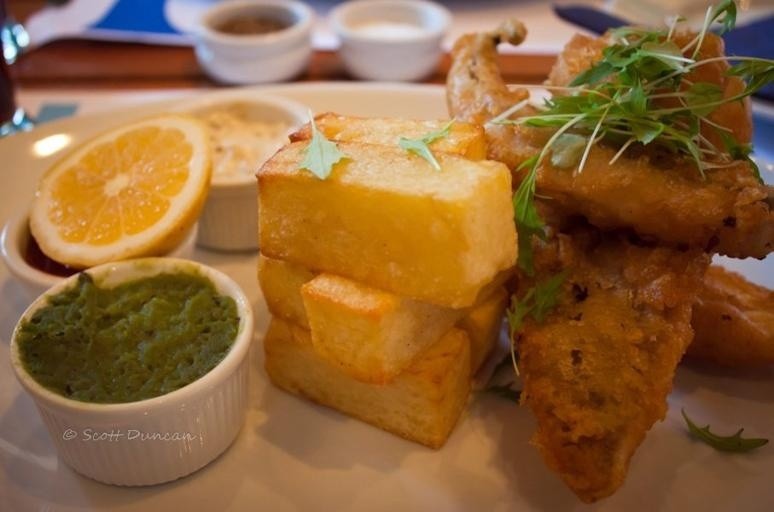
xmin=0 ymin=83 xmax=774 ymax=512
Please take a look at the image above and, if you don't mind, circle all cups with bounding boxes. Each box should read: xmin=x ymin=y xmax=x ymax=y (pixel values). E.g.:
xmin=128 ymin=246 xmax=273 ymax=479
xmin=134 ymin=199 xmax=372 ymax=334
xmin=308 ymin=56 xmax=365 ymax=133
xmin=331 ymin=1 xmax=457 ymax=79
xmin=187 ymin=4 xmax=319 ymax=82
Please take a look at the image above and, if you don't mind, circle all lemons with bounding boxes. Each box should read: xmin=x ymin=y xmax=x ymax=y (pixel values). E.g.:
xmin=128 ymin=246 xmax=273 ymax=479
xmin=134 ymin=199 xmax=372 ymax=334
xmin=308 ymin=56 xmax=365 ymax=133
xmin=29 ymin=112 xmax=213 ymax=267
